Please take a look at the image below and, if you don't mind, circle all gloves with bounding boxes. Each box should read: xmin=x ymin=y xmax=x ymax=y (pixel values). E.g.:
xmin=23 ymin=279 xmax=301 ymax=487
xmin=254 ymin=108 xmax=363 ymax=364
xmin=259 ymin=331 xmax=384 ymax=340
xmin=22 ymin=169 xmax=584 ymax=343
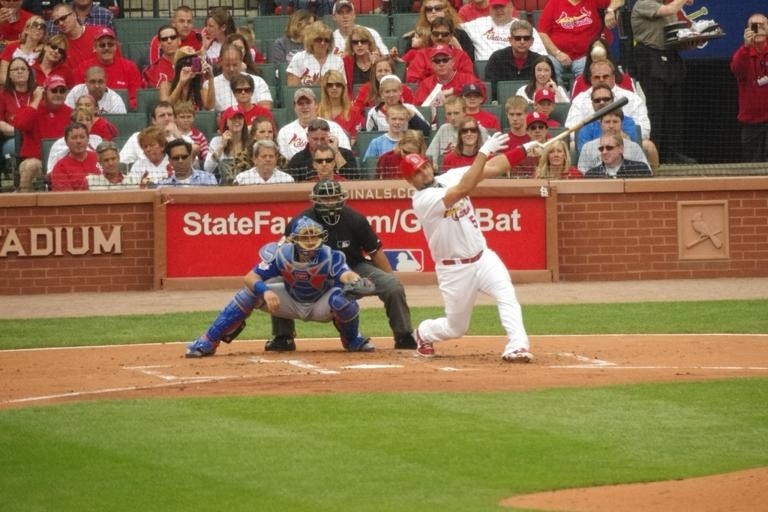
xmin=478 ymin=130 xmax=510 ymax=157
xmin=523 ymin=140 xmax=545 ymax=159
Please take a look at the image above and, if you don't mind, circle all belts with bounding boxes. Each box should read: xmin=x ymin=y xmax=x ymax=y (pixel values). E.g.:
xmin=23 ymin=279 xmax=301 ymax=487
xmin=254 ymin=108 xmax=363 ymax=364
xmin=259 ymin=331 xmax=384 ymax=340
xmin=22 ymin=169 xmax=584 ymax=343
xmin=437 ymin=250 xmax=492 ymax=266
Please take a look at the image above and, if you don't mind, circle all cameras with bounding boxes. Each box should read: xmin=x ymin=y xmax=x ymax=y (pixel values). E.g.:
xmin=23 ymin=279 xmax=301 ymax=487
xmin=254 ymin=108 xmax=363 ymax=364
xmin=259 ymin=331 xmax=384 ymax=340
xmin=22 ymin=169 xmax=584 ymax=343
xmin=192 ymin=57 xmax=201 ymax=72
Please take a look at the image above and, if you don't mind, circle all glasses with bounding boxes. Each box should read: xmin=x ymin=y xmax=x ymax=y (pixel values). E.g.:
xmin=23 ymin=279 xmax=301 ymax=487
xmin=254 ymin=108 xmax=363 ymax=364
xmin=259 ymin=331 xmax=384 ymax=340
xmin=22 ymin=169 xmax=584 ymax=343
xmin=591 ymin=96 xmax=612 ymax=103
xmin=324 ymin=82 xmax=344 ymax=89
xmin=313 ymin=36 xmax=331 ymax=44
xmin=54 ymin=12 xmax=73 ymax=26
xmin=530 ymin=125 xmax=544 ymax=130
xmin=461 ymin=127 xmax=477 ymax=134
xmin=234 ymin=87 xmax=252 ymax=94
xmin=171 ymin=154 xmax=189 ymax=162
xmin=593 ymin=74 xmax=610 ymax=81
xmin=30 ymin=21 xmax=47 ymax=31
xmin=314 ymin=158 xmax=334 ymax=164
xmin=160 ymin=34 xmax=177 ymax=41
xmin=99 ymin=42 xmax=114 ymax=49
xmin=47 ymin=42 xmax=65 ymax=55
xmin=351 ymin=38 xmax=369 ymax=45
xmin=51 ymin=87 xmax=67 ymax=94
xmin=512 ymin=35 xmax=532 ymax=41
xmin=424 ymin=5 xmax=446 ymax=13
xmin=598 ymin=145 xmax=614 ymax=152
xmin=432 ymin=57 xmax=452 ymax=64
xmin=431 ymin=30 xmax=451 ymax=37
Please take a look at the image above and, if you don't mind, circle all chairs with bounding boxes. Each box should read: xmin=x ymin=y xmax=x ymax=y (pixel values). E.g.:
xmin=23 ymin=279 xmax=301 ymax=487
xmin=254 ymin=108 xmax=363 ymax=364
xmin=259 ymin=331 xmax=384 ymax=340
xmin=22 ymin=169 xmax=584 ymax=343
xmin=0 ymin=1 xmax=650 ymax=196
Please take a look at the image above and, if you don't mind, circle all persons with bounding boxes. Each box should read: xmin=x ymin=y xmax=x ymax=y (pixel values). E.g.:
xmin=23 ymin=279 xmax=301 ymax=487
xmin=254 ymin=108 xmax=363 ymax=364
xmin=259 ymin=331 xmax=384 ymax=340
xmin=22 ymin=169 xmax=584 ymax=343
xmin=265 ymin=177 xmax=417 ymax=350
xmin=1 ymin=1 xmax=767 ymax=194
xmin=182 ymin=214 xmax=376 ymax=358
xmin=398 ymin=131 xmax=549 ymax=364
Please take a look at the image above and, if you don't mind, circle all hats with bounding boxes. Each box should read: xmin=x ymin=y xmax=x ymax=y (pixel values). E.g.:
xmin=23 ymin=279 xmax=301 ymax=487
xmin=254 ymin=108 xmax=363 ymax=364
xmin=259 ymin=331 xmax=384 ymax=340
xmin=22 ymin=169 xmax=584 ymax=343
xmin=223 ymin=105 xmax=245 ymax=119
xmin=398 ymin=152 xmax=429 ymax=177
xmin=462 ymin=82 xmax=483 ymax=97
xmin=534 ymin=88 xmax=555 ymax=103
xmin=94 ymin=28 xmax=116 ymax=41
xmin=526 ymin=111 xmax=548 ymax=125
xmin=293 ymin=86 xmax=317 ymax=104
xmin=332 ymin=0 xmax=354 ymax=15
xmin=173 ymin=46 xmax=199 ymax=65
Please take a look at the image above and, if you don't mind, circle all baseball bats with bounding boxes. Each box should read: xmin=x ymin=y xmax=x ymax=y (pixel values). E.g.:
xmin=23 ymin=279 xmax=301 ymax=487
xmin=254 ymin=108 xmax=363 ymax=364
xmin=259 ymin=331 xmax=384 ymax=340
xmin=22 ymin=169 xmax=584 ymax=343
xmin=530 ymin=96 xmax=628 ymax=158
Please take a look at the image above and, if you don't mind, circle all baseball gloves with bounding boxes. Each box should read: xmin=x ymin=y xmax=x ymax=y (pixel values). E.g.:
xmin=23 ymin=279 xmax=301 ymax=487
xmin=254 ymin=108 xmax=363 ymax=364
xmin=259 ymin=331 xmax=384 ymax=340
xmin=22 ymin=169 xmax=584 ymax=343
xmin=343 ymin=277 xmax=377 ymax=299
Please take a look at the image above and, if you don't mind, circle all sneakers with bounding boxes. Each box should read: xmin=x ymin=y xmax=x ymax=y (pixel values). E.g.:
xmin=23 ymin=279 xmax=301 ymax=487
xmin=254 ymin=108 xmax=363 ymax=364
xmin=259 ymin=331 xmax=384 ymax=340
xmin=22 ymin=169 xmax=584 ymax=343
xmin=394 ymin=332 xmax=416 ymax=350
xmin=185 ymin=343 xmax=216 ymax=358
xmin=342 ymin=337 xmax=376 ymax=352
xmin=504 ymin=347 xmax=533 ymax=362
xmin=266 ymin=335 xmax=296 ymax=351
xmin=414 ymin=327 xmax=436 ymax=360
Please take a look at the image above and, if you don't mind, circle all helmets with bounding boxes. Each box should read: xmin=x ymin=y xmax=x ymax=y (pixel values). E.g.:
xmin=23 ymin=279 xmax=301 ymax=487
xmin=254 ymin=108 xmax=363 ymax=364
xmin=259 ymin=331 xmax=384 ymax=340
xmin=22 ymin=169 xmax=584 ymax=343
xmin=289 ymin=216 xmax=328 ymax=258
xmin=311 ymin=179 xmax=350 ymax=218
